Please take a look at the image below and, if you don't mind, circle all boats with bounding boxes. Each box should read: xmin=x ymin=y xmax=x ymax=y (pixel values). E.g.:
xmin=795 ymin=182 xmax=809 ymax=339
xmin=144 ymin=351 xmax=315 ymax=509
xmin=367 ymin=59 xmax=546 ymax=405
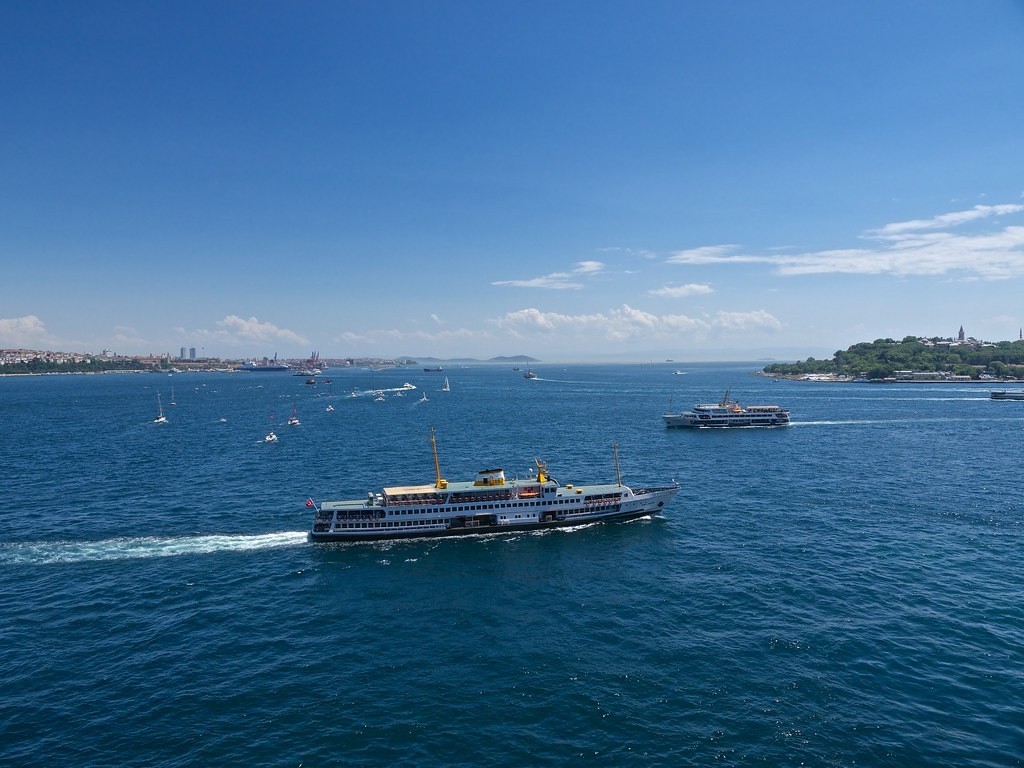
xmin=232 ymin=352 xmax=290 ymax=371
xmin=266 ymin=432 xmax=278 ymax=443
xmin=403 ymin=382 xmax=414 ymax=390
xmin=351 ymin=390 xmax=361 ymax=397
xmin=305 ymin=377 xmax=316 ymax=384
xmin=523 ymin=369 xmax=537 ymax=379
xmin=991 ymin=389 xmax=1024 ymax=400
xmin=394 ymin=391 xmax=402 ymax=397
xmin=423 ymin=367 xmax=444 ymax=372
xmin=305 ymin=425 xmax=684 ymax=540
xmin=661 ymin=384 xmax=792 ymax=427
xmin=288 ymin=417 xmax=300 ymax=425
xmin=369 ymin=389 xmax=386 ymax=401
xmin=513 ymin=366 xmax=520 ymax=371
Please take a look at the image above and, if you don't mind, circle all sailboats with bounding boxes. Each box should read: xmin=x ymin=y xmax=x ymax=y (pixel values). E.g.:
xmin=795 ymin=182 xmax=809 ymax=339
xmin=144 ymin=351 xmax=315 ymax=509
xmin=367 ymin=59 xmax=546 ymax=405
xmin=167 ymin=384 xmax=175 ymax=405
xmin=420 ymin=392 xmax=428 ymax=402
xmin=442 ymin=377 xmax=450 ymax=391
xmin=326 ymin=382 xmax=334 ymax=412
xmin=154 ymin=390 xmax=169 ymax=424
